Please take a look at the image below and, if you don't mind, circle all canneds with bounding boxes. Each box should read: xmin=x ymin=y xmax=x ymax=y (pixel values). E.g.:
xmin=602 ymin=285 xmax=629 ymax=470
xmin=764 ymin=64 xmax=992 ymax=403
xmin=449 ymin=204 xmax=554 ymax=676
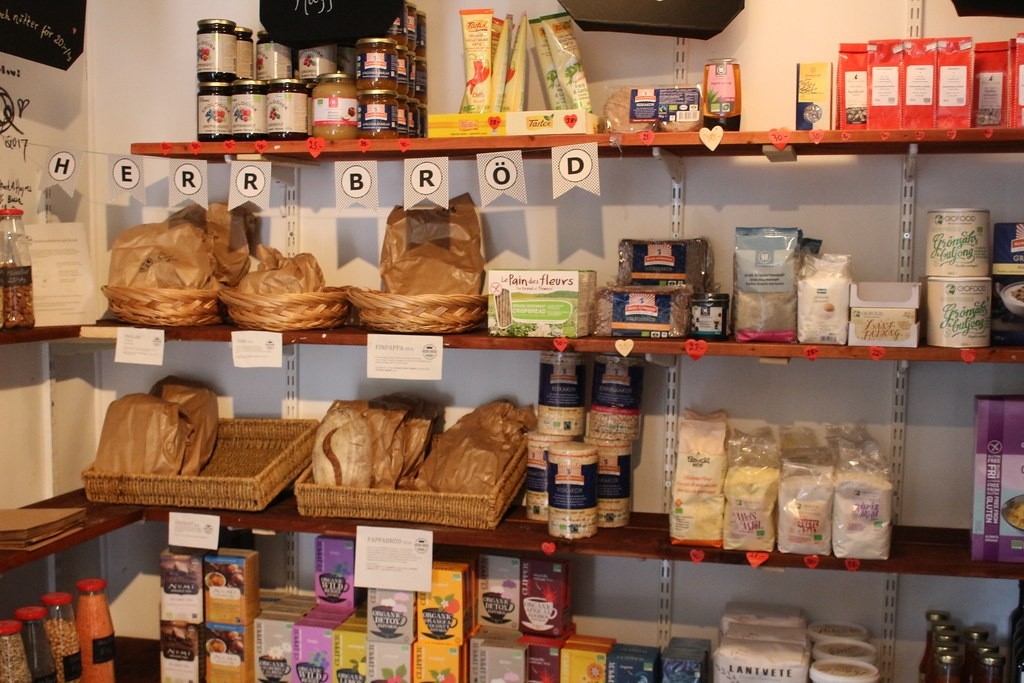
xmin=922 ymin=206 xmax=994 ymax=347
xmin=522 ymin=351 xmax=644 ymax=540
xmin=690 ymin=293 xmax=730 ymax=343
xmin=195 ymin=0 xmax=427 ymax=143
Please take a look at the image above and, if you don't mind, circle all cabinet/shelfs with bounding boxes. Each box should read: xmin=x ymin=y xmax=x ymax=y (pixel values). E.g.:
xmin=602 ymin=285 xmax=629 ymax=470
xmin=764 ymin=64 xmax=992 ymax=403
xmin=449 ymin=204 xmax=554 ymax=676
xmin=0 ymin=324 xmax=142 ymax=683
xmin=102 ymin=126 xmax=1024 ymax=683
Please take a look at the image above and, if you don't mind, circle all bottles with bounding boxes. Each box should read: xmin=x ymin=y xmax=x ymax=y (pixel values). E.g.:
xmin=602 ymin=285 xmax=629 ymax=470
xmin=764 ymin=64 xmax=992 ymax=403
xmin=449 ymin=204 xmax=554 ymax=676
xmin=918 ymin=610 xmax=1006 ymax=683
xmin=0 ymin=208 xmax=35 ymax=329
xmin=1009 ymin=579 xmax=1024 ymax=683
xmin=0 ymin=576 xmax=119 ymax=683
xmin=703 ymin=58 xmax=743 ymax=132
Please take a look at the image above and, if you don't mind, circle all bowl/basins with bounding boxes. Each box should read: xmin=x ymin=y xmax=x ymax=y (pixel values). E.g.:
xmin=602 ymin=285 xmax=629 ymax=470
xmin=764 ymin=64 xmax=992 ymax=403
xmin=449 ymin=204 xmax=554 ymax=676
xmin=1001 ymin=282 xmax=1024 ymax=315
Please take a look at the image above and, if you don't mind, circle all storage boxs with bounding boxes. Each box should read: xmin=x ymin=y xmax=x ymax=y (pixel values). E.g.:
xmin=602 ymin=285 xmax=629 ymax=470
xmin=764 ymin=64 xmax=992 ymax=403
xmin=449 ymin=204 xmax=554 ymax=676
xmin=253 ymin=613 xmax=300 ymax=683
xmin=796 ymin=62 xmax=833 ymax=131
xmin=318 ymin=605 xmax=357 ymax=618
xmin=160 ymin=549 xmax=205 ymax=624
xmin=343 ymin=618 xmax=368 ymax=627
xmin=367 ymin=642 xmax=415 ymax=683
xmin=274 ymin=600 xmax=316 ymax=611
xmin=262 ymin=605 xmax=307 ymax=618
xmin=204 ymin=623 xmax=254 ymax=683
xmin=333 ymin=625 xmax=367 ymax=683
xmin=284 ymin=594 xmax=315 ymax=603
xmin=293 ymin=620 xmax=339 ymax=683
xmin=488 ymin=269 xmax=597 ymax=338
xmin=367 ymin=586 xmax=416 ymax=642
xmin=969 ymin=393 xmax=1024 ymax=564
xmin=159 ymin=621 xmax=205 ymax=683
xmin=417 ymin=561 xmax=711 ymax=683
xmin=306 ymin=610 xmax=347 ymax=625
xmin=205 ymin=549 xmax=259 ymax=626
xmin=315 ymin=535 xmax=356 ymax=609
